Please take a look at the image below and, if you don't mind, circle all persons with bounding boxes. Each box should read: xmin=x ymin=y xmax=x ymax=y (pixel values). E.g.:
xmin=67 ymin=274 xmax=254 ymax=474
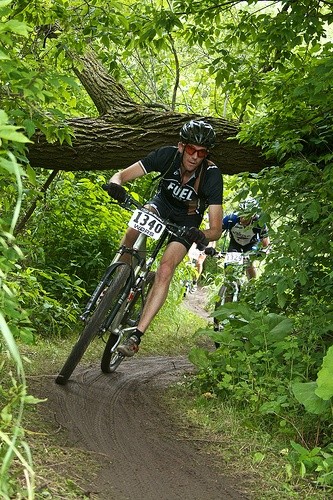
xmin=187 ymin=212 xmax=216 ymax=294
xmin=205 ymin=196 xmax=270 ymax=326
xmin=96 ymin=120 xmax=223 ymax=357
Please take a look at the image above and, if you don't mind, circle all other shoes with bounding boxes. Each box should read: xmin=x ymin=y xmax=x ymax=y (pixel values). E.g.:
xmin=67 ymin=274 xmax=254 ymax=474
xmin=117 ymin=335 xmax=139 ymax=356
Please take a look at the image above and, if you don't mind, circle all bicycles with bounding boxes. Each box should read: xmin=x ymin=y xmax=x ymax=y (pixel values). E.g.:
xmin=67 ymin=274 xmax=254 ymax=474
xmin=207 ymin=250 xmax=264 ymax=349
xmin=51 ymin=182 xmax=213 ymax=386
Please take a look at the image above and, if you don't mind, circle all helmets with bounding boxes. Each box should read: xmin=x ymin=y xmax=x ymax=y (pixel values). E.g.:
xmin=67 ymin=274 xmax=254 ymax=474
xmin=240 ymin=196 xmax=258 ymax=214
xmin=180 ymin=120 xmax=216 ymax=150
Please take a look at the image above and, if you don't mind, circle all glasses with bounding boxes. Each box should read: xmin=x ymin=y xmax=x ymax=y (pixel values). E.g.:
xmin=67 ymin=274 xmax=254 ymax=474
xmin=185 ymin=145 xmax=206 ymax=158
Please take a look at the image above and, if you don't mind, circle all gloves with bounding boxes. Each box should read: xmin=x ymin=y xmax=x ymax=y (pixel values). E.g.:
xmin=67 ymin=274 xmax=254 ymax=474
xmin=108 ymin=182 xmax=126 ymax=202
xmin=188 ymin=226 xmax=206 ymax=244
